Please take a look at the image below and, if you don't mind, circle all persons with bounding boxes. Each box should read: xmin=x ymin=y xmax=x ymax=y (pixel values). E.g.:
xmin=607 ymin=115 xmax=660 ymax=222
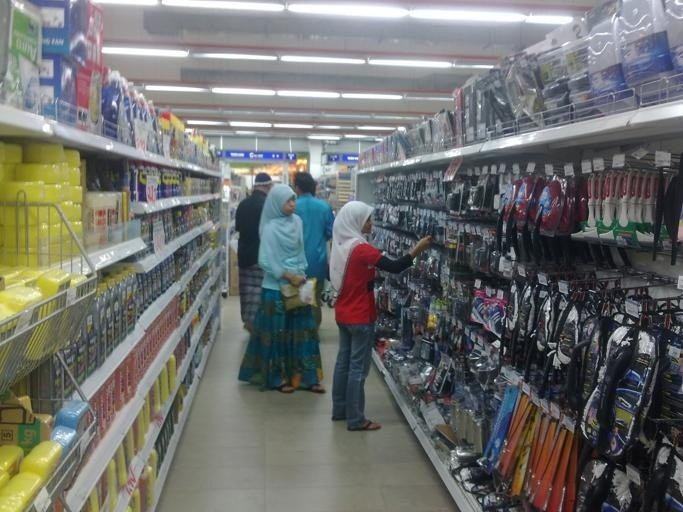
xmin=326 ymin=200 xmax=433 ymax=432
xmin=234 ymin=171 xmax=273 ymax=336
xmin=234 ymin=183 xmax=328 ymax=395
xmin=288 ymin=171 xmax=333 ymax=344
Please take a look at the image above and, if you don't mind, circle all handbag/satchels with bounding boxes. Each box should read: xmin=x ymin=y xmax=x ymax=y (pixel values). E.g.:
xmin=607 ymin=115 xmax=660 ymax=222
xmin=281 ymin=277 xmax=319 ymax=311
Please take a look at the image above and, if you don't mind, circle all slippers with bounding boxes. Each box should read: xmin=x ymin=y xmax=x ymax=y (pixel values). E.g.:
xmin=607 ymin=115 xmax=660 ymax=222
xmin=276 ymin=382 xmax=294 ymax=393
xmin=332 ymin=416 xmax=380 ymax=430
xmin=310 ymin=383 xmax=325 ymax=392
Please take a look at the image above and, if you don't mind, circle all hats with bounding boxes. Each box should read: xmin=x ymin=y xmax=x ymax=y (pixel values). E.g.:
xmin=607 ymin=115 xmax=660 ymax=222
xmin=255 ymin=173 xmax=272 ymax=185
